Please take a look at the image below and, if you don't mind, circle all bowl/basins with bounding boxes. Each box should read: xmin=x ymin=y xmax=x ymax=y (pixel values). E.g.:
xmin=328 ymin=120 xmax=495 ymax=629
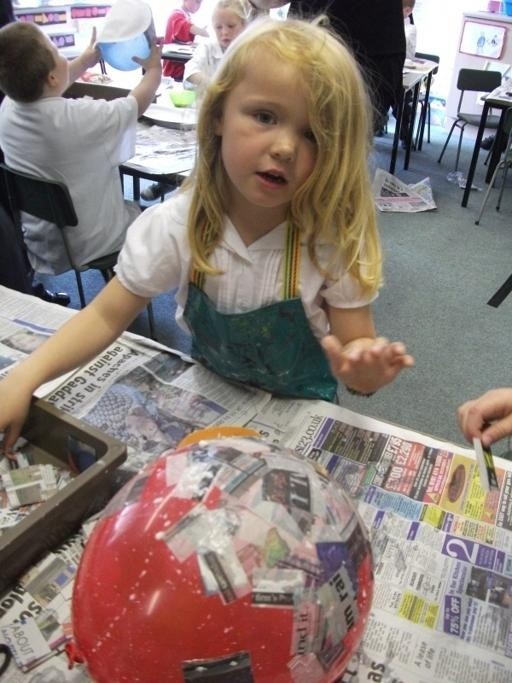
xmin=171 ymin=90 xmax=195 ymax=106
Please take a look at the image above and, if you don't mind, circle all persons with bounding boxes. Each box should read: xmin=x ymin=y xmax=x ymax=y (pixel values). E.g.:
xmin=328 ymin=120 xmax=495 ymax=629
xmin=0 ymin=21 xmax=173 ymax=308
xmin=455 ymin=388 xmax=511 ymax=449
xmin=162 ymin=0 xmax=209 ymax=85
xmin=0 ymin=21 xmax=414 ymax=458
xmin=181 ymin=1 xmax=415 ymax=148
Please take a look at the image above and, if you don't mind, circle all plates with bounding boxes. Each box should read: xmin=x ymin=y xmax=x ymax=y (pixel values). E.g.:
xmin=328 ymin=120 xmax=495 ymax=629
xmin=141 ymin=102 xmax=199 ymax=127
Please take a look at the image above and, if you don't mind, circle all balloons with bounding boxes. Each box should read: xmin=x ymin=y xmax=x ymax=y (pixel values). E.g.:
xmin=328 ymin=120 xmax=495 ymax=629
xmin=64 ymin=436 xmax=373 ymax=683
xmin=92 ymin=0 xmax=153 ymax=71
xmin=169 ymin=87 xmax=196 ymax=107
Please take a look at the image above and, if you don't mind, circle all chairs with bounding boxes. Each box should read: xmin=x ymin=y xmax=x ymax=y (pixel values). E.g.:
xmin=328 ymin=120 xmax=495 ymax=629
xmin=438 ymin=67 xmax=511 ymax=224
xmin=412 ymin=51 xmax=442 ymax=149
xmin=0 ymin=163 xmax=158 ymax=341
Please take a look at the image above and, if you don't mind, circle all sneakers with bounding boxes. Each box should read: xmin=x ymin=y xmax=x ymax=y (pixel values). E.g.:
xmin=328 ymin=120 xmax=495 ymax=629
xmin=480 ymin=134 xmax=508 ymax=153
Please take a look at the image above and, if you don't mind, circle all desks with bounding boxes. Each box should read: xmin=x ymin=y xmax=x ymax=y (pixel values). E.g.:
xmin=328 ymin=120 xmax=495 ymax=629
xmin=158 ymin=41 xmax=439 ymax=176
xmin=118 ymin=81 xmax=200 ymax=210
